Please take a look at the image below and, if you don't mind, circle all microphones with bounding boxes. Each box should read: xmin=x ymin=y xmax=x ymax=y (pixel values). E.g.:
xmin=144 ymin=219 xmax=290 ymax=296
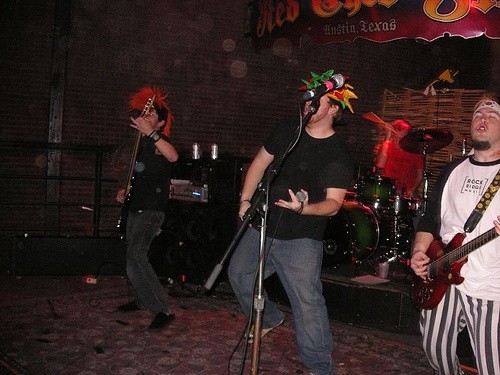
xmin=302 ymin=74 xmax=345 ymax=102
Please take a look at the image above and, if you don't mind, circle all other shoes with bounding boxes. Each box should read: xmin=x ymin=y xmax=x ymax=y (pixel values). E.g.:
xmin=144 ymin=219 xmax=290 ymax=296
xmin=118 ymin=302 xmax=139 ymax=312
xmin=149 ymin=312 xmax=175 ymax=332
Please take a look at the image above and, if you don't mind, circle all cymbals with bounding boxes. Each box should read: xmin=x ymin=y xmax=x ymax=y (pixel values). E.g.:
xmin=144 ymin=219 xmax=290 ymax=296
xmin=399 ymin=128 xmax=454 ymax=155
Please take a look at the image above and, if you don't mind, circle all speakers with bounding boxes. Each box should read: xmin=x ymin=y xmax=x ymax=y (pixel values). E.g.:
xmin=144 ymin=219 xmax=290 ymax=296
xmin=320 ymin=217 xmax=348 ymax=263
xmin=150 ymin=205 xmax=238 ymax=285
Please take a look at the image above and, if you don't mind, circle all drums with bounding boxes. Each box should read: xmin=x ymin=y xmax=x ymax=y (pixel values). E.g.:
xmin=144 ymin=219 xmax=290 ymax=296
xmin=380 ymin=223 xmax=409 ymax=249
xmin=385 ymin=195 xmax=420 ymax=215
xmin=323 ymin=200 xmax=380 ymax=267
xmin=356 ymin=174 xmax=397 ymax=209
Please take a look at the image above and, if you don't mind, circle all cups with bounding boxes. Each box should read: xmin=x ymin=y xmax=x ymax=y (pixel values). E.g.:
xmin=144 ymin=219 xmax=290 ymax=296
xmin=379 ymin=262 xmax=389 ymax=279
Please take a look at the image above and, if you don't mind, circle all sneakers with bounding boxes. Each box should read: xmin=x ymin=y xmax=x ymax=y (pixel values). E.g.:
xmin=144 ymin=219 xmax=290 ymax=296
xmin=244 ymin=317 xmax=285 ymax=343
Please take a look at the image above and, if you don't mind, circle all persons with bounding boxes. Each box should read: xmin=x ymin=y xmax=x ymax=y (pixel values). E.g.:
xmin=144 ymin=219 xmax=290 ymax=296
xmin=373 ymin=120 xmax=424 ymax=274
xmin=228 ymin=68 xmax=358 ymax=375
xmin=410 ymin=93 xmax=500 ymax=375
xmin=116 ymin=84 xmax=178 ymax=333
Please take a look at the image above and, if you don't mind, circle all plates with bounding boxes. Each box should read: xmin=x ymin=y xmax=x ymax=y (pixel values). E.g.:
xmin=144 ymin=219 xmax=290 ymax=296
xmin=349 ymin=275 xmax=390 ymax=285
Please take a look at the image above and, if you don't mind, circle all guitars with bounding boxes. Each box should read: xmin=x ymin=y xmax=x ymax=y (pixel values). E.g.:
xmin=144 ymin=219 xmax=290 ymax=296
xmin=411 ymin=223 xmax=500 ymax=309
xmin=116 ymin=93 xmax=158 ymax=233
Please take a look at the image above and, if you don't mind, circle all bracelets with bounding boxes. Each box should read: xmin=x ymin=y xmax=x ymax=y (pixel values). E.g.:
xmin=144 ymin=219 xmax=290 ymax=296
xmin=239 ymin=200 xmax=250 ymax=204
xmin=296 ymin=202 xmax=303 ymax=215
xmin=149 ymin=130 xmax=161 ymax=144
